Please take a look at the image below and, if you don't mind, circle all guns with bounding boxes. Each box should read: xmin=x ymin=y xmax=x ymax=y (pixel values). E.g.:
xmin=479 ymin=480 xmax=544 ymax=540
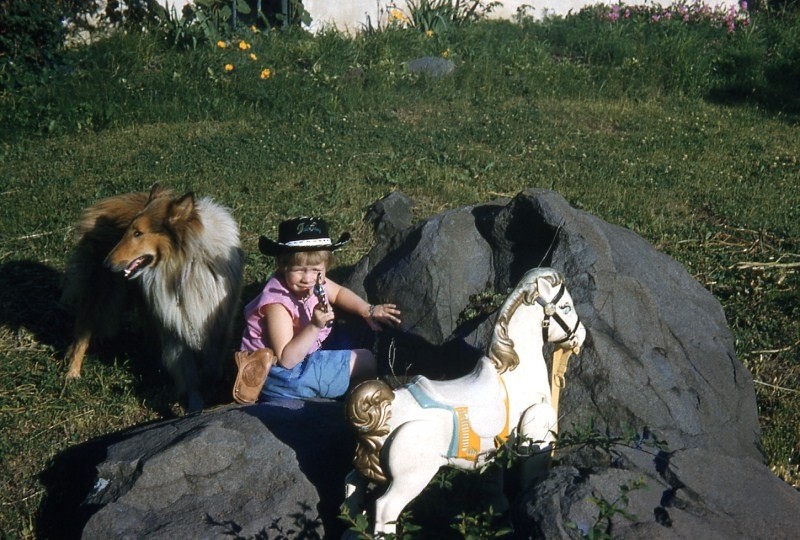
xmin=315 ymin=273 xmax=336 ymax=329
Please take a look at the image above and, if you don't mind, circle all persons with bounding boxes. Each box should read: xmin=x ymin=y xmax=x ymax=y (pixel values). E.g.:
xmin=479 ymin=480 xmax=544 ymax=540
xmin=240 ymin=217 xmax=401 ymax=400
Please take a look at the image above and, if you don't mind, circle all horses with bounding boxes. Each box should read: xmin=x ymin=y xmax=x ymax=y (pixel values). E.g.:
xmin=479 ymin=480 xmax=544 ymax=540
xmin=340 ymin=266 xmax=587 ymax=540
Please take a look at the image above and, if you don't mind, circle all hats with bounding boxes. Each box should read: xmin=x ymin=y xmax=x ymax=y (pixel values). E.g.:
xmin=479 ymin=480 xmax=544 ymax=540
xmin=259 ymin=217 xmax=351 ymax=257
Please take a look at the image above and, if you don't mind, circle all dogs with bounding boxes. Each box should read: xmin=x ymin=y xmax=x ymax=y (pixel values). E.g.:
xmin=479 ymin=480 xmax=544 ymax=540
xmin=62 ymin=181 xmax=245 ymax=414
